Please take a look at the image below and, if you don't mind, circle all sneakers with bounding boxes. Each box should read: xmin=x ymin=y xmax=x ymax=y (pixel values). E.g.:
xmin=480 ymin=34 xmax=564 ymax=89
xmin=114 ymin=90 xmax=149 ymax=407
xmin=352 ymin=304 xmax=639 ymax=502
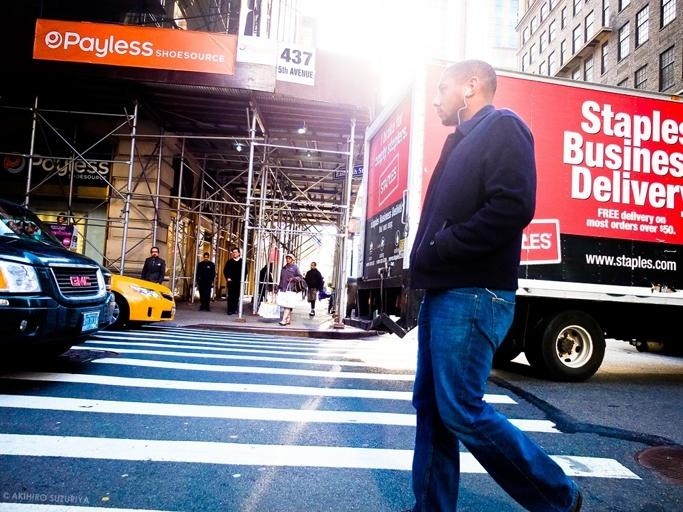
xmin=309 ymin=311 xmax=315 ymax=316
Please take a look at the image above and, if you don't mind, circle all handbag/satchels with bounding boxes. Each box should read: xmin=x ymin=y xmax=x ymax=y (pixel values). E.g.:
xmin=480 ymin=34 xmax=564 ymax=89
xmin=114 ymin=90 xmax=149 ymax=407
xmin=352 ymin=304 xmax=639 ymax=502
xmin=276 ymin=290 xmax=303 ymax=309
xmin=256 ymin=302 xmax=281 ymax=319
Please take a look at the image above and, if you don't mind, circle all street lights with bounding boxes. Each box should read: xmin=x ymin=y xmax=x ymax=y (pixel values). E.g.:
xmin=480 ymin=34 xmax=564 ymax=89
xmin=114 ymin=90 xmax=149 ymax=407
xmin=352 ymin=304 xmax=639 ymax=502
xmin=347 ymin=216 xmax=361 ymax=276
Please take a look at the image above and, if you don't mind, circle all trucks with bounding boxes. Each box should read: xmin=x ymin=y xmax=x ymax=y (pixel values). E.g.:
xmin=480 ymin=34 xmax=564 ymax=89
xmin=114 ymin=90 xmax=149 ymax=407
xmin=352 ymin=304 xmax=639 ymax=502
xmin=342 ymin=60 xmax=683 ymax=383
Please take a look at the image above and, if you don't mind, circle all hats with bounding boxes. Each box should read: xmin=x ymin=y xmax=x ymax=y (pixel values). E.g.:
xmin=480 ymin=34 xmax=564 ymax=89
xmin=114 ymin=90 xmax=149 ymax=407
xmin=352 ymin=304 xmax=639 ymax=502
xmin=285 ymin=254 xmax=295 ymax=261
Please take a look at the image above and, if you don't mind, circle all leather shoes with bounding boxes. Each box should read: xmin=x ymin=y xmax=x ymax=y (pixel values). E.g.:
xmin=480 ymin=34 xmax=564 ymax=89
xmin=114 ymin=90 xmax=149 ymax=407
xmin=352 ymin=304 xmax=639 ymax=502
xmin=279 ymin=321 xmax=290 ymax=326
xmin=227 ymin=310 xmax=238 ymax=315
xmin=198 ymin=307 xmax=211 ymax=312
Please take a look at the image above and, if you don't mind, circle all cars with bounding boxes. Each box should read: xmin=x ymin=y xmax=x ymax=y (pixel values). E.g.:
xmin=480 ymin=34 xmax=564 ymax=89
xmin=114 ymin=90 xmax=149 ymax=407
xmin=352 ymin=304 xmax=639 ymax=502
xmin=0 ymin=198 xmax=176 ymax=356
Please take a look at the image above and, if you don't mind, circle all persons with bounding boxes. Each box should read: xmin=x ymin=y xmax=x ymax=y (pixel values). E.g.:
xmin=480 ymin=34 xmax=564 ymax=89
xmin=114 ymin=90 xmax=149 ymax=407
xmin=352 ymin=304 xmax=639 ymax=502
xmin=223 ymin=248 xmax=247 ymax=315
xmin=403 ymin=59 xmax=588 ymax=512
xmin=299 ymin=273 xmax=308 ymax=299
xmin=139 ymin=247 xmax=165 ymax=284
xmin=303 ymin=262 xmax=322 ymax=317
xmin=274 ymin=254 xmax=302 ymax=327
xmin=193 ymin=251 xmax=215 ymax=312
